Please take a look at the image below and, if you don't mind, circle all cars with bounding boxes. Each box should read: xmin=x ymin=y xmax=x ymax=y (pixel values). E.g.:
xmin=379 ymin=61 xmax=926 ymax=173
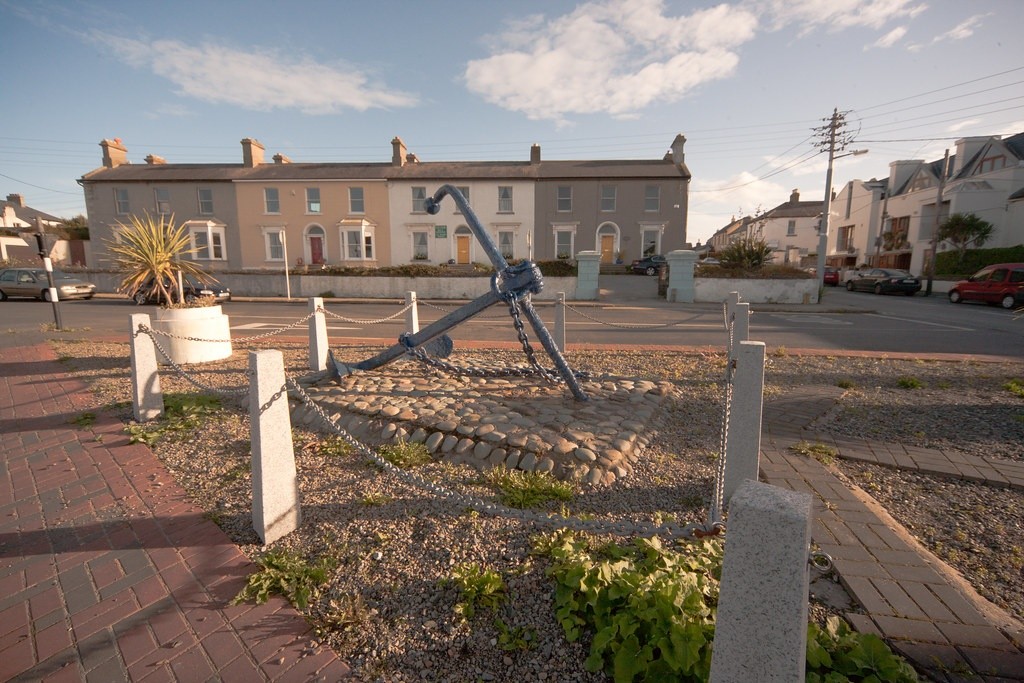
xmin=703 ymin=258 xmax=720 ymax=265
xmin=0 ymin=267 xmax=97 ymax=304
xmin=631 ymin=254 xmax=667 ymax=276
xmin=129 ymin=274 xmax=231 ymax=306
xmin=810 ymin=266 xmax=839 ymax=287
xmin=846 ymin=268 xmax=922 ymax=297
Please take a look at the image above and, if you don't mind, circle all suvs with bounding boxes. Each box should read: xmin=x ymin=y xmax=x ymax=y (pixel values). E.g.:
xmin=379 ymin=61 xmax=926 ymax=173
xmin=947 ymin=263 xmax=1024 ymax=310
